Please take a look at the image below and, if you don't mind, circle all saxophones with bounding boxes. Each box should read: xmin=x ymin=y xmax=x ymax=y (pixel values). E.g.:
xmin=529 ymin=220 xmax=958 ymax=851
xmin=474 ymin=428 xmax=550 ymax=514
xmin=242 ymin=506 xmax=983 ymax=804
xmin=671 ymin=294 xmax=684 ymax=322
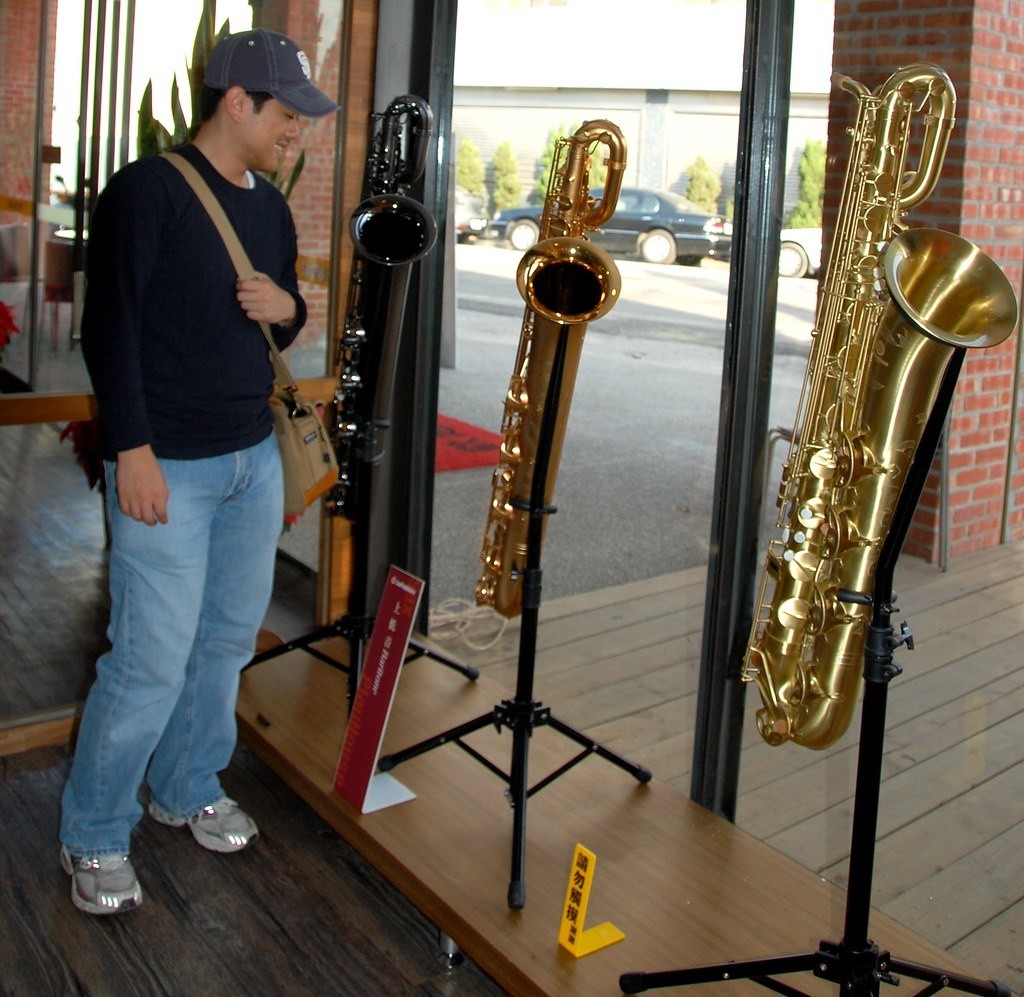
xmin=736 ymin=62 xmax=1021 ymax=755
xmin=471 ymin=118 xmax=629 ymax=622
xmin=319 ymin=92 xmax=441 ymax=522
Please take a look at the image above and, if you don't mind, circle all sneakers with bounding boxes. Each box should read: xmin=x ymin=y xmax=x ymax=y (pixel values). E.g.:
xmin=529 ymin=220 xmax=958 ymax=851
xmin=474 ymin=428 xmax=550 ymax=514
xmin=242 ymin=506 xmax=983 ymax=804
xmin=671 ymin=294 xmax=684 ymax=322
xmin=148 ymin=789 xmax=259 ymax=853
xmin=60 ymin=841 xmax=143 ymax=915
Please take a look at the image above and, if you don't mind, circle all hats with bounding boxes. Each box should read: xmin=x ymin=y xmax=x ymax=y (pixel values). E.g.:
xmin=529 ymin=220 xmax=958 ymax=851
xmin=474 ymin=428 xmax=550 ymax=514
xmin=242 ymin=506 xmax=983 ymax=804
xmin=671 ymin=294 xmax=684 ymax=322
xmin=202 ymin=29 xmax=340 ymax=118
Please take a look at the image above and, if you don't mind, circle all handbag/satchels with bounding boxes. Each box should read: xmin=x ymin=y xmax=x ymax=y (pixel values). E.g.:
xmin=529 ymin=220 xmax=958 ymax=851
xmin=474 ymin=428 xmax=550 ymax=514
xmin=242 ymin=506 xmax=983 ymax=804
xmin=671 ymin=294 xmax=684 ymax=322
xmin=270 ymin=387 xmax=339 ymax=517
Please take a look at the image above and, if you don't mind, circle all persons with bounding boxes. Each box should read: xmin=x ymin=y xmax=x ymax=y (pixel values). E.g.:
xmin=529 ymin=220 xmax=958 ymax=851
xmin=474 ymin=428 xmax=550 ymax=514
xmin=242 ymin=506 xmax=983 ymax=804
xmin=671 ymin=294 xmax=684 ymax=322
xmin=59 ymin=28 xmax=341 ymax=916
